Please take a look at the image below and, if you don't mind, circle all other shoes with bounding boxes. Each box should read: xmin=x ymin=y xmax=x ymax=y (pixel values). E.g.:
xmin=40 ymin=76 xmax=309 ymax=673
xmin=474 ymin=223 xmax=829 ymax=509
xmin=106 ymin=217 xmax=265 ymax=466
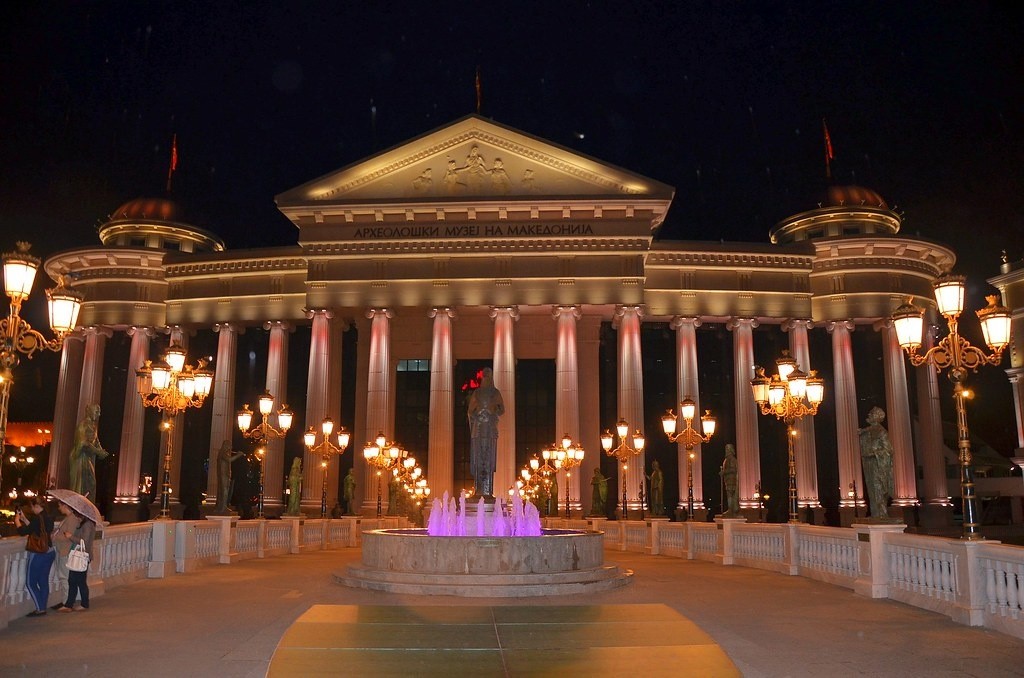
xmin=26 ymin=610 xmax=46 ymax=617
xmin=75 ymin=605 xmax=86 ymax=611
xmin=57 ymin=605 xmax=73 ymax=612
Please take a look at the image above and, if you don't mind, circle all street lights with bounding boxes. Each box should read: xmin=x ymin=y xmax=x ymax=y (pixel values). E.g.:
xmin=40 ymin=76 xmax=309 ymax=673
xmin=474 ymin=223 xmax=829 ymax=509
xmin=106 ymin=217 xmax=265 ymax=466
xmin=750 ymin=346 xmax=825 ymax=521
xmin=133 ymin=337 xmax=215 ymax=520
xmin=0 ymin=238 xmax=86 ymax=475
xmin=509 ymin=443 xmax=563 ymax=517
xmin=237 ymin=390 xmax=294 ymax=520
xmin=659 ymin=394 xmax=717 ymax=522
xmin=302 ymin=416 xmax=352 ymax=519
xmin=886 ymin=269 xmax=1014 ymax=541
xmin=599 ymin=418 xmax=646 ymax=521
xmin=9 ymin=444 xmax=35 ymax=505
xmin=363 ymin=431 xmax=400 ymax=519
xmin=391 ymin=452 xmax=430 ymax=527
xmin=556 ymin=432 xmax=586 ymax=519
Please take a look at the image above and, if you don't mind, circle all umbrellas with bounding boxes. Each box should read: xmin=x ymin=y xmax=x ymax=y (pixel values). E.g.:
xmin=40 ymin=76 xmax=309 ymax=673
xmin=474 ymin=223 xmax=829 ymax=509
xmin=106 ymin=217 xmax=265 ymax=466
xmin=47 ymin=489 xmax=103 ymax=536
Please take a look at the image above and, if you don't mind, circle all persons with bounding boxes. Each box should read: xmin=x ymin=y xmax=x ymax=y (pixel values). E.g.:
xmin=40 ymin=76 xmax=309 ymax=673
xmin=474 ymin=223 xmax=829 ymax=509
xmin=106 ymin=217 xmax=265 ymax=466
xmin=13 ymin=499 xmax=56 ymax=614
xmin=49 ymin=501 xmax=82 ymax=610
xmin=643 ymin=460 xmax=665 ymax=516
xmin=212 ymin=439 xmax=244 ymax=511
xmin=589 ymin=466 xmax=607 ymax=515
xmin=550 ymin=474 xmax=559 ymax=516
xmin=386 ymin=477 xmax=397 ymax=514
xmin=57 ymin=509 xmax=96 ymax=612
xmin=285 ymin=457 xmax=306 ymax=516
xmin=68 ymin=401 xmax=109 ymax=503
xmin=719 ymin=443 xmax=743 ymax=516
xmin=857 ymin=407 xmax=894 ymax=517
xmin=343 ymin=469 xmax=358 ymax=516
xmin=467 ymin=368 xmax=505 ymax=504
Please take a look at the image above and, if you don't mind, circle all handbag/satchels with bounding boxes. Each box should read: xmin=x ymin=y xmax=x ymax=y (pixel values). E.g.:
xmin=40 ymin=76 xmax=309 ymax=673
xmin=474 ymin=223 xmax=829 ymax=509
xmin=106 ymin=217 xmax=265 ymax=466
xmin=25 ymin=515 xmax=48 ymax=553
xmin=65 ymin=539 xmax=89 ymax=572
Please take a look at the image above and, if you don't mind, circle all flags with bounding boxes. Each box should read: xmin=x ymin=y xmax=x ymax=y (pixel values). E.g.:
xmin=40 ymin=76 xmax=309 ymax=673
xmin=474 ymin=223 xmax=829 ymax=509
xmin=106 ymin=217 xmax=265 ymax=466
xmin=821 ymin=123 xmax=837 ymax=164
xmin=475 ymin=70 xmax=481 ymax=110
xmin=167 ymin=135 xmax=179 ymax=179
